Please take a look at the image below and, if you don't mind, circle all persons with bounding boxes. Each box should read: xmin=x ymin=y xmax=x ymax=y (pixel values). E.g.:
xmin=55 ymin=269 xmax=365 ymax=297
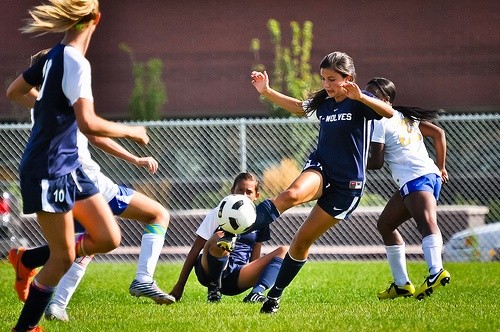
xmin=366 ymin=78 xmax=451 ymax=300
xmin=0 ymin=0 xmax=149 ymax=332
xmin=44 ymin=118 xmax=176 ymax=322
xmin=168 ymin=173 xmax=288 ymax=304
xmin=217 ymin=53 xmax=394 ymax=310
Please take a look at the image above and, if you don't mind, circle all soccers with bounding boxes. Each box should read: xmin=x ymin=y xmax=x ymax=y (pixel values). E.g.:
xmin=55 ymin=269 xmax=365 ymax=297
xmin=217 ymin=194 xmax=257 ymax=234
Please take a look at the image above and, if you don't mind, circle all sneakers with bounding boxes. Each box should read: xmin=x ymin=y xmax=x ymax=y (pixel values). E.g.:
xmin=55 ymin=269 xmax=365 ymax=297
xmin=260 ymin=296 xmax=281 ymax=314
xmin=129 ymin=280 xmax=175 ymax=305
xmin=217 ymin=232 xmax=237 ymax=252
xmin=208 ymin=288 xmax=220 ymax=302
xmin=413 ymin=270 xmax=450 ymax=301
xmin=378 ymin=281 xmax=415 ymax=299
xmin=9 ymin=248 xmax=34 ymax=302
xmin=244 ymin=293 xmax=266 ymax=302
xmin=45 ymin=301 xmax=69 ymax=321
xmin=12 ymin=326 xmax=44 ymax=332
